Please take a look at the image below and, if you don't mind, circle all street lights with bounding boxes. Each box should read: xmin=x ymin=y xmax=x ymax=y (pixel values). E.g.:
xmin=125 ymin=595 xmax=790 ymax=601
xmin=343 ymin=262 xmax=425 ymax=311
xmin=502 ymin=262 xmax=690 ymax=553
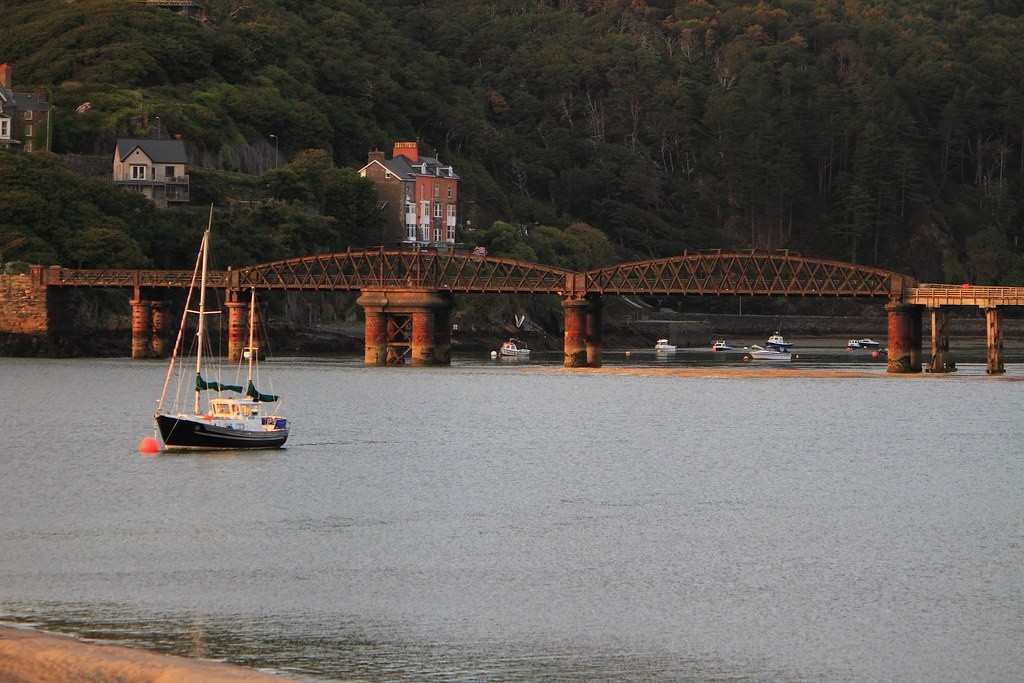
xmin=156 ymin=116 xmax=160 ymax=139
xmin=270 ymin=135 xmax=278 ymax=168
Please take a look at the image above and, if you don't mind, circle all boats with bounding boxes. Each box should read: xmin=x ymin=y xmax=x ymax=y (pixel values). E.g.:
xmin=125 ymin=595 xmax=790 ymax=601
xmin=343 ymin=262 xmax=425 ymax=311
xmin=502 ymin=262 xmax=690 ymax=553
xmin=765 ymin=328 xmax=793 ymax=349
xmin=859 ymin=337 xmax=880 ymax=347
xmin=500 ymin=338 xmax=530 ymax=356
xmin=749 ymin=344 xmax=791 ymax=360
xmin=847 ymin=338 xmax=862 ymax=348
xmin=713 ymin=341 xmax=731 ymax=350
xmin=655 ymin=338 xmax=676 ymax=351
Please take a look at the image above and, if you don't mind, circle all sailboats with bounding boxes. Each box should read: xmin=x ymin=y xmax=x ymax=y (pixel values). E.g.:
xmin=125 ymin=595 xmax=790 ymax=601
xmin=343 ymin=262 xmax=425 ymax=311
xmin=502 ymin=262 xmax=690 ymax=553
xmin=154 ymin=202 xmax=292 ymax=452
